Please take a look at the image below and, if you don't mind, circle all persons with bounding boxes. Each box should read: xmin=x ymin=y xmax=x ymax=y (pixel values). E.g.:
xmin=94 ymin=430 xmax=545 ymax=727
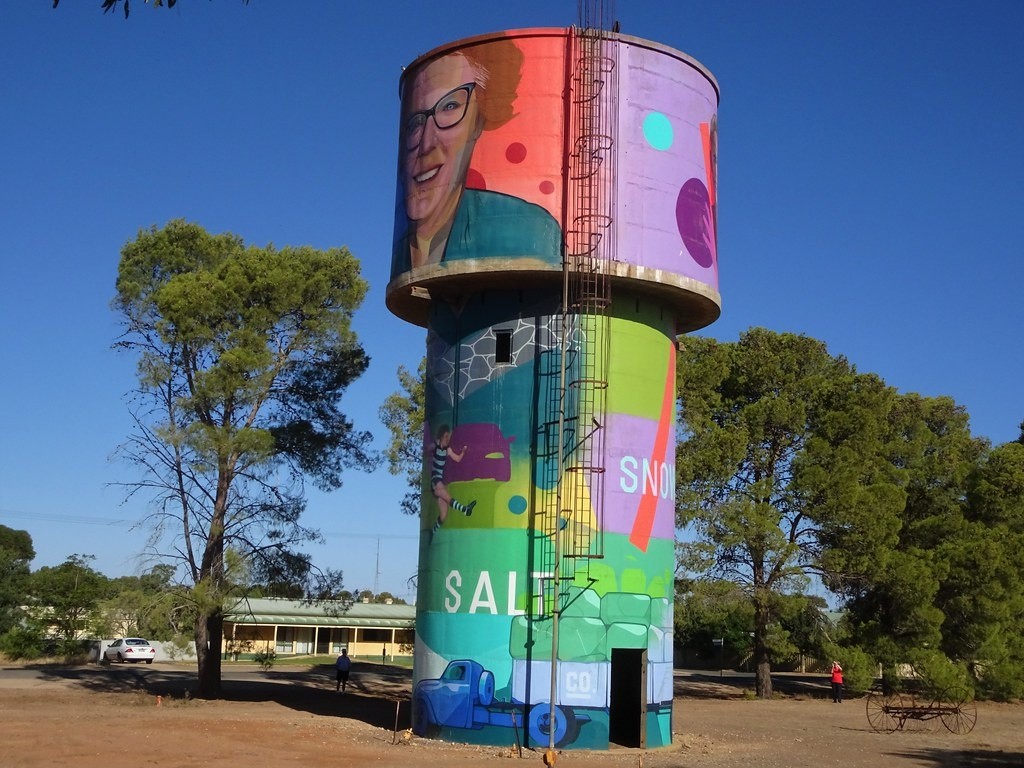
xmin=831 ymin=661 xmax=843 ymax=703
xmin=335 ymin=649 xmax=352 ymax=693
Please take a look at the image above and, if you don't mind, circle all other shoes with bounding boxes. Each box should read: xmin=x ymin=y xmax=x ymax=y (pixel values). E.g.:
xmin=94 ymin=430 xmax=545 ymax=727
xmin=838 ymin=699 xmax=841 ymax=703
xmin=832 ymin=699 xmax=837 ymax=703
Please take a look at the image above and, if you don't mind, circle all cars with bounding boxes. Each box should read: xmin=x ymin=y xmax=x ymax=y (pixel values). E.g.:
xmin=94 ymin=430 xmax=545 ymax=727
xmin=102 ymin=637 xmax=156 ymax=664
